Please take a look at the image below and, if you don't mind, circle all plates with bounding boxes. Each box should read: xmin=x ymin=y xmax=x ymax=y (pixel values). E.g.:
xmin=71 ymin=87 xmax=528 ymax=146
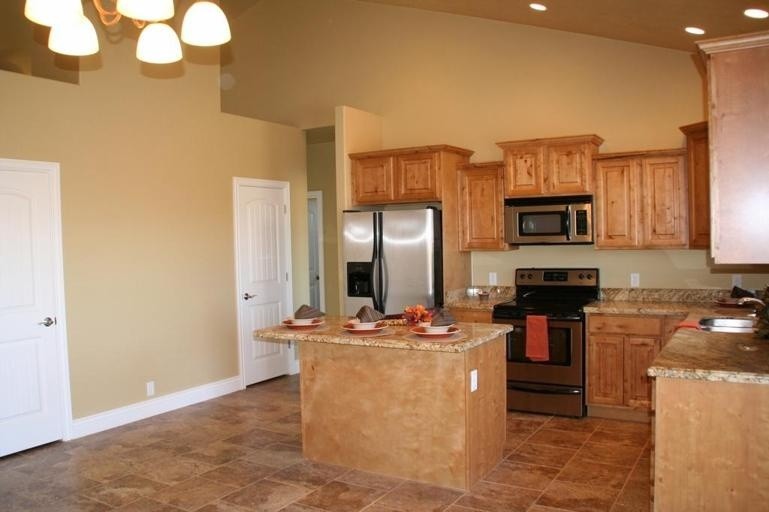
xmin=341 ymin=324 xmax=388 ymax=333
xmin=410 ymin=327 xmax=462 ymax=336
xmin=281 ymin=321 xmax=325 ymax=327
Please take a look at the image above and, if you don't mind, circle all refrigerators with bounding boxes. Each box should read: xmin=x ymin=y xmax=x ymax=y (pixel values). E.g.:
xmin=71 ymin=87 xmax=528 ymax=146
xmin=340 ymin=209 xmax=441 ymax=318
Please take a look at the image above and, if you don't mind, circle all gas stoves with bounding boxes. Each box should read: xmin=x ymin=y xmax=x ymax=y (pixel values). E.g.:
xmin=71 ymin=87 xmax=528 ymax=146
xmin=492 ymin=286 xmax=602 ymax=321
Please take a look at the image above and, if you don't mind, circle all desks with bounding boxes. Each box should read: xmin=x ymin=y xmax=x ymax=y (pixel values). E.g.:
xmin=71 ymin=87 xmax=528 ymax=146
xmin=251 ymin=314 xmax=516 ymax=493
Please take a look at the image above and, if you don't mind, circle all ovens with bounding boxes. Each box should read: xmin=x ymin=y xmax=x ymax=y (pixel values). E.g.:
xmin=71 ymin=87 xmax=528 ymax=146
xmin=497 ymin=321 xmax=585 ymax=417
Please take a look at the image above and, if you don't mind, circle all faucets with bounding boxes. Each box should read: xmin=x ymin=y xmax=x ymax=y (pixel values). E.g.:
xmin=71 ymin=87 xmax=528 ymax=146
xmin=737 ymin=296 xmax=767 ymax=309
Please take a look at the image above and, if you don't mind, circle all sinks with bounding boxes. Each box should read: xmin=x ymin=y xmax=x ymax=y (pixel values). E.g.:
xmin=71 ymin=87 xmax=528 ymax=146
xmin=699 ymin=326 xmax=755 ymax=334
xmin=698 ymin=317 xmax=754 ymax=328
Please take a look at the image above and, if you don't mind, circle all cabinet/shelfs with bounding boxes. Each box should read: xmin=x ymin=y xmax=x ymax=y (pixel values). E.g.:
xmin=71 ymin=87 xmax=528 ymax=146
xmin=678 ymin=120 xmax=712 ymax=250
xmin=454 ymin=162 xmax=518 ymax=252
xmin=449 ymin=296 xmax=514 ymax=323
xmin=591 ymin=148 xmax=691 ymax=251
xmin=695 ymin=27 xmax=769 ymax=271
xmin=649 ymin=303 xmax=768 ymax=512
xmin=346 ymin=144 xmax=474 ymax=204
xmin=495 ymin=133 xmax=606 ymax=196
xmin=584 ymin=302 xmax=663 ymax=421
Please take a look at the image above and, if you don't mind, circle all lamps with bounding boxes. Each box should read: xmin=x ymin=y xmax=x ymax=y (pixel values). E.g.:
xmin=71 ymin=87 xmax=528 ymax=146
xmin=19 ymin=0 xmax=234 ymax=69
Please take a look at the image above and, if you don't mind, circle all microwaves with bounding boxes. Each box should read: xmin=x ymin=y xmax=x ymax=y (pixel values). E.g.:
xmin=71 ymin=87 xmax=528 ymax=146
xmin=504 ymin=204 xmax=592 ymax=246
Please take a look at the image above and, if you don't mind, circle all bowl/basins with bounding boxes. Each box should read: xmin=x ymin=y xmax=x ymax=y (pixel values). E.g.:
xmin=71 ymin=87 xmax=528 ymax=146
xmin=347 ymin=318 xmax=382 ymax=329
xmin=288 ymin=315 xmax=319 ymax=324
xmin=419 ymin=320 xmax=455 ymax=333
